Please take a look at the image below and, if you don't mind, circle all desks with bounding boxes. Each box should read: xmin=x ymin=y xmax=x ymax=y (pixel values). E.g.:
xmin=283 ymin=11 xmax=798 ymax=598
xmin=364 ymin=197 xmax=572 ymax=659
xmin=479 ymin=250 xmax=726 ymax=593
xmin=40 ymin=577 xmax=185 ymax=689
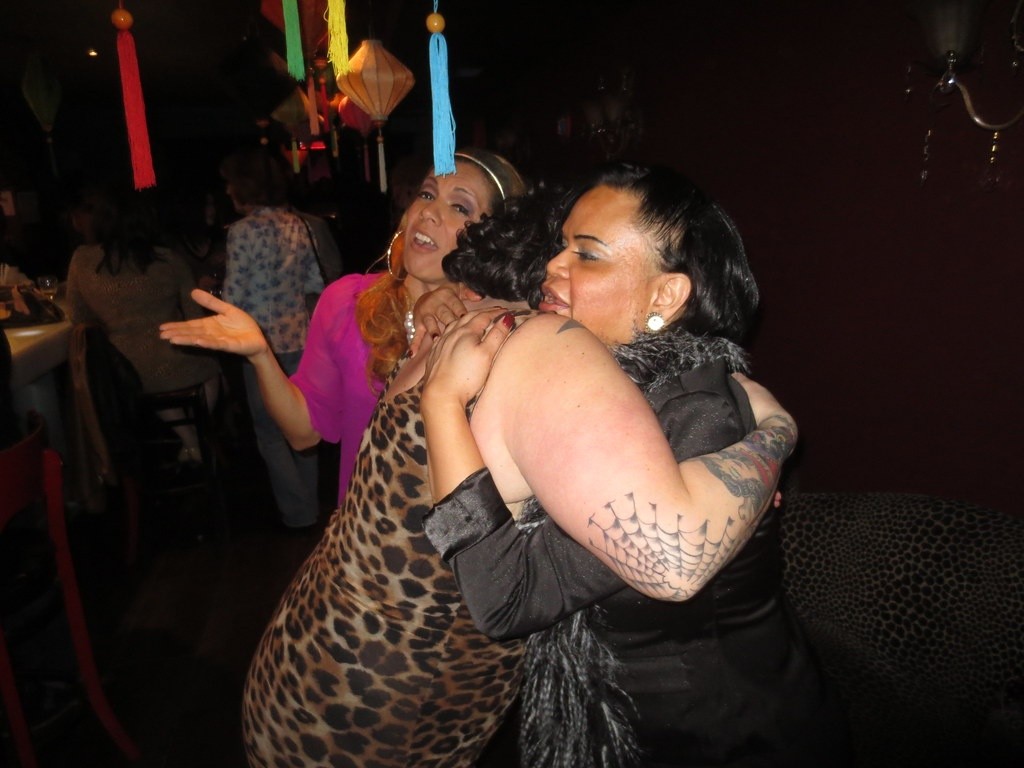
xmin=2 ymin=321 xmax=75 ymax=473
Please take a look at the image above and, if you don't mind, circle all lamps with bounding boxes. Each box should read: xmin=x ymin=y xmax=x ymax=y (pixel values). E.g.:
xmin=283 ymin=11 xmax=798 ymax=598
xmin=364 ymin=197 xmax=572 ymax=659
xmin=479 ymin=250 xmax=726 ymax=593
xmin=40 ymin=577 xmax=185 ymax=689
xmin=892 ymin=0 xmax=1024 ymax=190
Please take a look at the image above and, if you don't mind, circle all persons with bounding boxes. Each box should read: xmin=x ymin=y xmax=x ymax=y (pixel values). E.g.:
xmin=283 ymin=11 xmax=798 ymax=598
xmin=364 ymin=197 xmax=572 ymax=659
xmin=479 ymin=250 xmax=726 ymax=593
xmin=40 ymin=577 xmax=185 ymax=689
xmin=157 ymin=144 xmax=858 ymax=768
xmin=0 ymin=183 xmax=229 ymax=473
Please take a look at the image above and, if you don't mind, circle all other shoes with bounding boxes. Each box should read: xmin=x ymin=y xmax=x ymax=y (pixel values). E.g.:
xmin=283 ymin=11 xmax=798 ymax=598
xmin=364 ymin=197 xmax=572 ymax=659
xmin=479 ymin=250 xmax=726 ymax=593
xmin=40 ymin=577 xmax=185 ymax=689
xmin=176 ymin=446 xmax=202 ymax=463
xmin=278 ymin=523 xmax=316 ymax=538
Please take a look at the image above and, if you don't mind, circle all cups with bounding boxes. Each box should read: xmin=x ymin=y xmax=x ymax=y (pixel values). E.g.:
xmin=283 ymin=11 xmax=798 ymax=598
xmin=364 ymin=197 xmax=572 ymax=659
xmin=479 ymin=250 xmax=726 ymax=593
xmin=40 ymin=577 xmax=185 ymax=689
xmin=37 ymin=277 xmax=58 ymax=303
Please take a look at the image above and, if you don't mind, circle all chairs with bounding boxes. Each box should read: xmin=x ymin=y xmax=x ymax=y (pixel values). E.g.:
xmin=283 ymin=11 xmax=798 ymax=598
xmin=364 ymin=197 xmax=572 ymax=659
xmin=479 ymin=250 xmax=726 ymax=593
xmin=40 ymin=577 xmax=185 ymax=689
xmin=0 ymin=409 xmax=148 ymax=768
xmin=84 ymin=327 xmax=226 ymax=585
